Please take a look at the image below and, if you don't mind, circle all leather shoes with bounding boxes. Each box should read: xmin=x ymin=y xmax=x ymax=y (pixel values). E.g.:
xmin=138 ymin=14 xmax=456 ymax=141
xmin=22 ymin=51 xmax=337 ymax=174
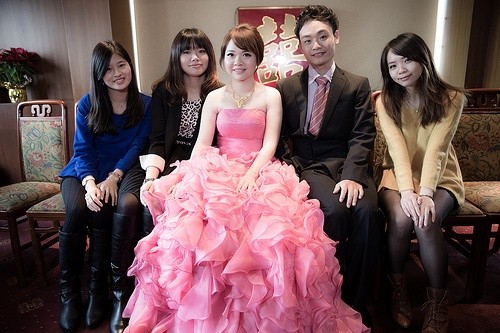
xmin=353 ymin=304 xmax=372 ymax=324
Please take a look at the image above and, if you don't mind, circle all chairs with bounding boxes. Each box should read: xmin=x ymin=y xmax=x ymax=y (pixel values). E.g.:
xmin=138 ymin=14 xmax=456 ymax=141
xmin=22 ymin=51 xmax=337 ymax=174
xmin=0 ymin=99 xmax=79 ymax=279
xmin=371 ymin=88 xmax=500 ymax=299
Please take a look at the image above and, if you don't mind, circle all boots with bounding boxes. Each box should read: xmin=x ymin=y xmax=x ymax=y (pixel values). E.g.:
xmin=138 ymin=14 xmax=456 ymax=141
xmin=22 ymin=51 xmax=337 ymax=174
xmin=85 ymin=227 xmax=112 ymax=329
xmin=59 ymin=225 xmax=88 ymax=331
xmin=387 ymin=271 xmax=412 ymax=328
xmin=137 ymin=212 xmax=154 ymax=239
xmin=419 ymin=287 xmax=449 ymax=333
xmin=110 ymin=213 xmax=139 ymax=333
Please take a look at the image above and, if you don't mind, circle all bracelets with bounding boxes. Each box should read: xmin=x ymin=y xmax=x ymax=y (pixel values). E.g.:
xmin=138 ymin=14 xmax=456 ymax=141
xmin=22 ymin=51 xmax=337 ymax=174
xmin=144 ymin=178 xmax=155 ymax=183
xmin=108 ymin=171 xmax=122 ymax=183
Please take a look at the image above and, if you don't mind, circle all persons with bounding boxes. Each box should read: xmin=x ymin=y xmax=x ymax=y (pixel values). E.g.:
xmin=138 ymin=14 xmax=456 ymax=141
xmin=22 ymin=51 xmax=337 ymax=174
xmin=121 ymin=23 xmax=371 ymax=333
xmin=372 ymin=32 xmax=468 ymax=333
xmin=109 ymin=28 xmax=226 ymax=333
xmin=56 ymin=40 xmax=152 ymax=331
xmin=274 ymin=4 xmax=379 ymax=333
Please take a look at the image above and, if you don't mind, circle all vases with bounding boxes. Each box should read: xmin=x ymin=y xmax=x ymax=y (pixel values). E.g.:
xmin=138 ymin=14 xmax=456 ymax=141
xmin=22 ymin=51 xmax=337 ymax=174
xmin=8 ymin=85 xmax=27 ymax=103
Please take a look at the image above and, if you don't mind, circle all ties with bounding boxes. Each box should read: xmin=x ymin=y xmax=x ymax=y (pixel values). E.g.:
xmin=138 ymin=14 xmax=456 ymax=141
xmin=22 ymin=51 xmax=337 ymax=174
xmin=308 ymin=76 xmax=328 ymax=136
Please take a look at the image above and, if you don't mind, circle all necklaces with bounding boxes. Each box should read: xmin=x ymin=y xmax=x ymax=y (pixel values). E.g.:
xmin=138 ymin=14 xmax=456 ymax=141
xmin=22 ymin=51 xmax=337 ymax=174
xmin=230 ymin=81 xmax=255 ymax=107
xmin=404 ymin=96 xmax=420 ymax=112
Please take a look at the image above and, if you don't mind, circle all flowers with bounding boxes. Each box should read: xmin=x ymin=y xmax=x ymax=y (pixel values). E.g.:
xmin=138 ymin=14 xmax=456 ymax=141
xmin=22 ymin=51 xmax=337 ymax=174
xmin=0 ymin=48 xmax=44 ymax=88
